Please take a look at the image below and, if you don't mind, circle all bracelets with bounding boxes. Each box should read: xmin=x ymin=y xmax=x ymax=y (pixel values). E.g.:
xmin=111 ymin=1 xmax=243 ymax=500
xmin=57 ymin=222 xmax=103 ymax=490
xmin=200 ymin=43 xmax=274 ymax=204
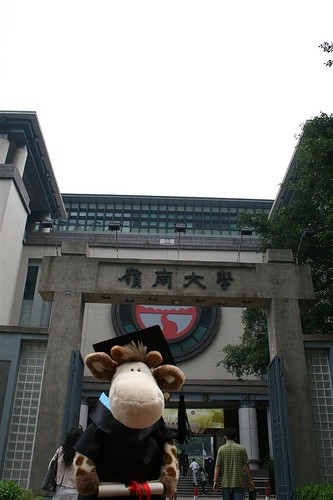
xmin=214 ymin=480 xmax=218 ymax=482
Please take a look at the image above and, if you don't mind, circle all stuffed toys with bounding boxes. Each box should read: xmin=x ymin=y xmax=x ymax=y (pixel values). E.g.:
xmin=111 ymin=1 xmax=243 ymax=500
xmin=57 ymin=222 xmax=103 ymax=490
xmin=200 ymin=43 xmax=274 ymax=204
xmin=72 ymin=325 xmax=186 ymax=500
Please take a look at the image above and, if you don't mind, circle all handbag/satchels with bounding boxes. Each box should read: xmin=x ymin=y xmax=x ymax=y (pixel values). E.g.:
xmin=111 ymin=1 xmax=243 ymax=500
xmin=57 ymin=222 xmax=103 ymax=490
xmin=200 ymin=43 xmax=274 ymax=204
xmin=41 ymin=447 xmax=59 ymax=492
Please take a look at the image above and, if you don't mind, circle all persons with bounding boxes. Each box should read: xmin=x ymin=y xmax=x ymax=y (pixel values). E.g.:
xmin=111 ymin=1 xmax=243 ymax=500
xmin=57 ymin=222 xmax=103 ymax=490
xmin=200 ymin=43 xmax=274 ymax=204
xmin=179 ymin=450 xmax=214 ymax=500
xmin=213 ymin=428 xmax=255 ymax=500
xmin=48 ymin=424 xmax=83 ymax=500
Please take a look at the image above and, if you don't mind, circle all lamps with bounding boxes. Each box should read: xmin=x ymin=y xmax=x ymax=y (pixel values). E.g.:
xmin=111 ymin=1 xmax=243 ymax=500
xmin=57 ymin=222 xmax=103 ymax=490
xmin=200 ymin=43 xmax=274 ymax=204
xmin=108 ymin=222 xmax=121 ymax=258
xmin=40 ymin=219 xmax=59 ymax=256
xmin=236 ymin=227 xmax=252 ymax=263
xmin=174 ymin=223 xmax=187 ymax=260
xmin=295 ymin=228 xmax=314 ymax=265
